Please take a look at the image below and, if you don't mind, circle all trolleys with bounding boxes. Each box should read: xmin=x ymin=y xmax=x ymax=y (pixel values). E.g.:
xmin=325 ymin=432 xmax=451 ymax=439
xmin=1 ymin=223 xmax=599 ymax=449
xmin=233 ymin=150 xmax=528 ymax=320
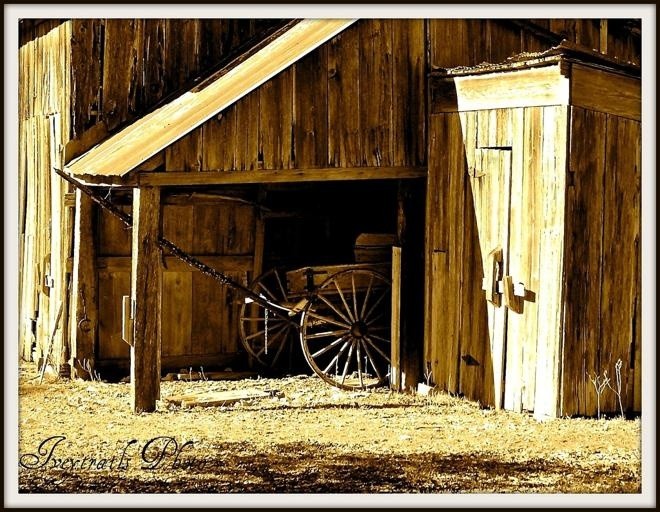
xmin=238 ymin=259 xmax=391 ymax=393
